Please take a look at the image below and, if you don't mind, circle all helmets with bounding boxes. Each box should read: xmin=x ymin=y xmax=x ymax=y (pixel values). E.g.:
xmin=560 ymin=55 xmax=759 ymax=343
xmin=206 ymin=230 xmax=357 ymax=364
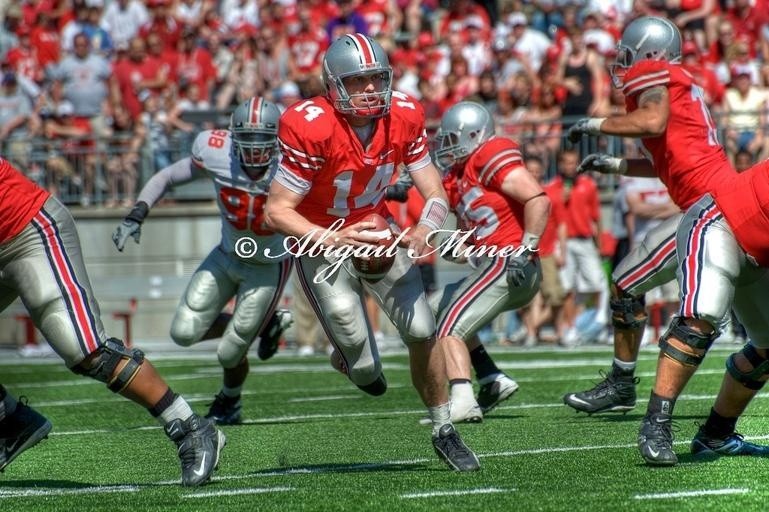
xmin=610 ymin=15 xmax=684 ymax=87
xmin=323 ymin=33 xmax=393 ymax=119
xmin=229 ymin=97 xmax=281 ymax=169
xmin=434 ymin=102 xmax=495 ymax=171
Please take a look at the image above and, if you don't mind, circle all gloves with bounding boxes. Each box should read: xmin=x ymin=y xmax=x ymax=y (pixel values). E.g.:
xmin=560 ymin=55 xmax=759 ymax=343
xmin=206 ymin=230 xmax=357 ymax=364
xmin=506 ymin=244 xmax=535 ymax=287
xmin=568 ymin=115 xmax=607 ymax=143
xmin=113 ymin=217 xmax=141 ymax=252
xmin=577 ymin=154 xmax=627 ymax=176
xmin=440 ymin=237 xmax=470 ymax=264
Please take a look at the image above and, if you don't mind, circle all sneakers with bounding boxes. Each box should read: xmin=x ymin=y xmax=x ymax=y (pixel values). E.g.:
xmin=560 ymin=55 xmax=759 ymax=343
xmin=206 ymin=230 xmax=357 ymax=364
xmin=259 ymin=310 xmax=293 ymax=360
xmin=432 ymin=425 xmax=480 ymax=473
xmin=476 ymin=373 xmax=518 ymax=411
xmin=420 ymin=400 xmax=483 ymax=425
xmin=203 ymin=391 xmax=242 ymax=422
xmin=165 ymin=413 xmax=226 ymax=488
xmin=563 ymin=361 xmax=640 ymax=415
xmin=639 ymin=413 xmax=681 ymax=464
xmin=691 ymin=420 xmax=769 ymax=458
xmin=331 ymin=350 xmax=387 ymax=396
xmin=0 ymin=394 xmax=52 ymax=472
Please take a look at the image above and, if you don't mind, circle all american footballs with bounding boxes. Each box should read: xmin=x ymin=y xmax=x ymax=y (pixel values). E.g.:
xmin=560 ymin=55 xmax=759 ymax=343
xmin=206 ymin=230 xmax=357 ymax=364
xmin=351 ymin=214 xmax=397 ymax=285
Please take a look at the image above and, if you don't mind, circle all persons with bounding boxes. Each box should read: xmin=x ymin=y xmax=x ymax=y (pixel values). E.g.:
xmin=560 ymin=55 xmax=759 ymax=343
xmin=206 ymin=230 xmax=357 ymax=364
xmin=0 ymin=0 xmax=769 ymax=512
